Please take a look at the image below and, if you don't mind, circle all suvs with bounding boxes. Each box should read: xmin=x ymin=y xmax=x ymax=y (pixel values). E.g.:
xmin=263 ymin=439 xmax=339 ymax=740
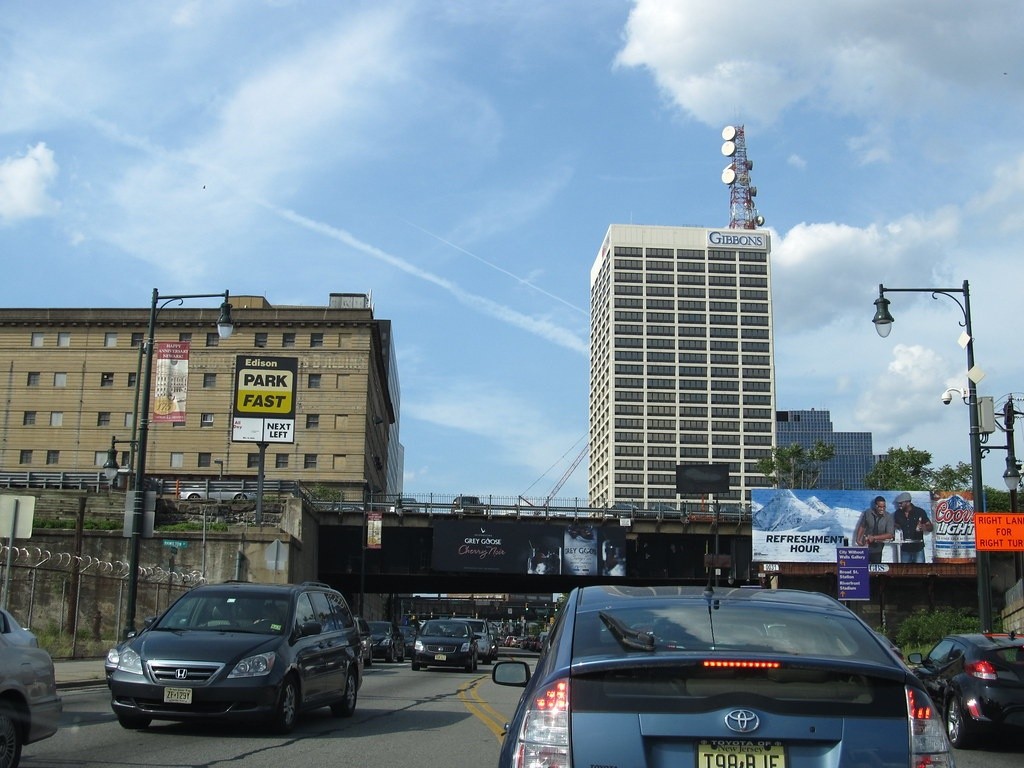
xmin=104 ymin=579 xmax=363 ymax=736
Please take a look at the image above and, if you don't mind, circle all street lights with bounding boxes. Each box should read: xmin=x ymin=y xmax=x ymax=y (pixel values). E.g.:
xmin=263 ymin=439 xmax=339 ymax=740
xmin=102 ymin=287 xmax=233 ymax=644
xmin=872 ymin=280 xmax=1021 ymax=633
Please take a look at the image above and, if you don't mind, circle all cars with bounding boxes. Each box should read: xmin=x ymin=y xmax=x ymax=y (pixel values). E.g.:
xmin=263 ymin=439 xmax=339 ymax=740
xmin=491 ymin=583 xmax=955 ymax=767
xmin=353 ymin=617 xmax=374 ymax=665
xmin=490 ymin=634 xmax=501 ymax=660
xmin=0 ymin=608 xmax=62 ymax=768
xmin=366 ymin=621 xmax=406 ymax=663
xmin=644 ymin=505 xmax=681 ymax=519
xmin=443 ymin=618 xmax=492 ymax=665
xmin=611 ymin=504 xmax=644 ymax=517
xmin=397 ymin=626 xmax=417 ymax=656
xmin=178 ymin=488 xmax=258 ymax=500
xmin=504 ymin=632 xmax=548 ymax=652
xmin=451 ymin=496 xmax=484 ymax=514
xmin=907 ymin=633 xmax=1023 ymax=750
xmin=412 ymin=619 xmax=481 ymax=671
xmin=390 ymin=498 xmax=420 ymax=513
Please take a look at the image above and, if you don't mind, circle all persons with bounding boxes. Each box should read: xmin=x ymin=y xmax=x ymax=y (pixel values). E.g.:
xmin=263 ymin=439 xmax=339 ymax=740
xmin=857 ymin=496 xmax=895 ymax=564
xmin=895 ymin=493 xmax=934 ymax=564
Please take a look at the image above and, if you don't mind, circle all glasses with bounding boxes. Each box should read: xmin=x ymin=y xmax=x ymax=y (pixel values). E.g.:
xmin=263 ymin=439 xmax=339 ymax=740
xmin=876 ymin=504 xmax=886 ymax=509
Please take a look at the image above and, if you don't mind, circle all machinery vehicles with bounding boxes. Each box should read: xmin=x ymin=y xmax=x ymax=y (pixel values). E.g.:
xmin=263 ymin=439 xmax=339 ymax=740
xmin=518 ymin=431 xmax=589 ymax=516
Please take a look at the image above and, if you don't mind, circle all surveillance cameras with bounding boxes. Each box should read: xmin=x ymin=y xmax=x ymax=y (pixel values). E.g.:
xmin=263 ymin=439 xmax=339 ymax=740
xmin=941 ymin=391 xmax=952 ymax=405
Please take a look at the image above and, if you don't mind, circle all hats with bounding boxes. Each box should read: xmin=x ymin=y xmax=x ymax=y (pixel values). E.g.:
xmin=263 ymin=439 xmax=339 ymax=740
xmin=894 ymin=492 xmax=911 ymax=502
